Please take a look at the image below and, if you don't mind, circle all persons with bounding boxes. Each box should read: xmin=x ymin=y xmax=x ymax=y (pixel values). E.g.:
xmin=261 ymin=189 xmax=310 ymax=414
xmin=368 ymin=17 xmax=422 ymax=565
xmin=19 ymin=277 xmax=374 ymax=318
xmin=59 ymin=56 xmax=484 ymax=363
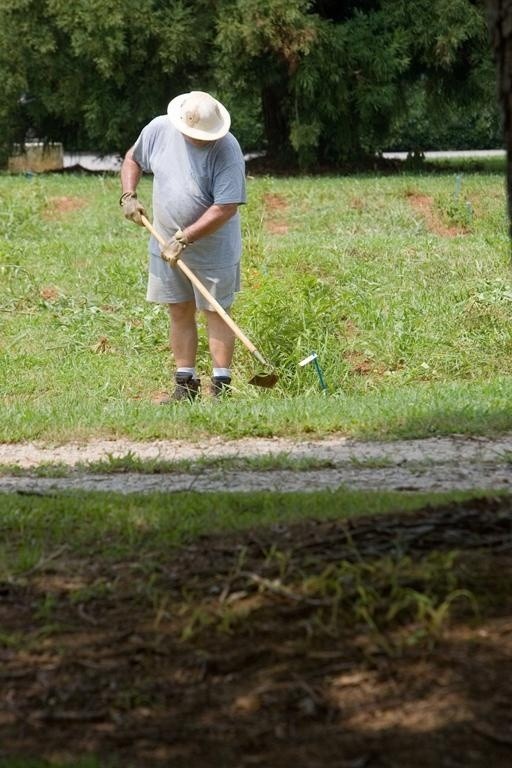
xmin=119 ymin=90 xmax=248 ymax=408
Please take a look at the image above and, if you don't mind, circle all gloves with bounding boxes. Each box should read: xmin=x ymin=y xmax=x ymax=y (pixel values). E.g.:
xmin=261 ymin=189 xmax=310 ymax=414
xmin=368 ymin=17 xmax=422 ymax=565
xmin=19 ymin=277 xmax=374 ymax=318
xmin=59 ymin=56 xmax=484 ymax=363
xmin=161 ymin=230 xmax=193 ymax=265
xmin=119 ymin=192 xmax=149 ymax=227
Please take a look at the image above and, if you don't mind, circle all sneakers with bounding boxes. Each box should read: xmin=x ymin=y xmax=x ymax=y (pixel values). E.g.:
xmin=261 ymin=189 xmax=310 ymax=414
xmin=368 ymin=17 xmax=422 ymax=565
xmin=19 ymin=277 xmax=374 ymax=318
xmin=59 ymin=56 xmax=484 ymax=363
xmin=160 ymin=372 xmax=200 ymax=405
xmin=210 ymin=376 xmax=231 ymax=403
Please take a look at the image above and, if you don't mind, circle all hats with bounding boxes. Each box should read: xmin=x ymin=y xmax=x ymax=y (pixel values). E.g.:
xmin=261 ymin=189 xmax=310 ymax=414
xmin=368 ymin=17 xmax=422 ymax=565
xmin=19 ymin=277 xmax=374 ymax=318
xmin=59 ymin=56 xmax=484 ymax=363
xmin=167 ymin=91 xmax=231 ymax=141
